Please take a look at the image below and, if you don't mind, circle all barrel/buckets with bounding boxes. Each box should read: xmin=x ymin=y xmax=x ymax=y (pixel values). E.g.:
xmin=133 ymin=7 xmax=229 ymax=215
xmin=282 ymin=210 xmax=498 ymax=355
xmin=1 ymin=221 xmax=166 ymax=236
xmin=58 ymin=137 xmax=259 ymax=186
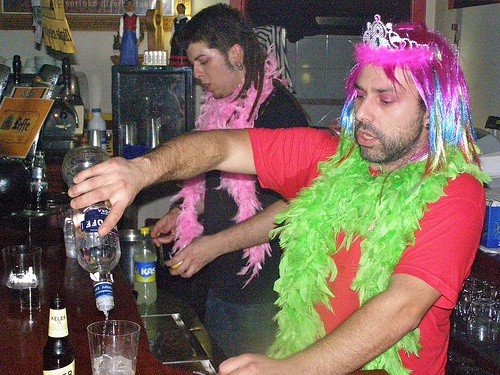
xmin=119 ymin=227 xmax=140 ymax=293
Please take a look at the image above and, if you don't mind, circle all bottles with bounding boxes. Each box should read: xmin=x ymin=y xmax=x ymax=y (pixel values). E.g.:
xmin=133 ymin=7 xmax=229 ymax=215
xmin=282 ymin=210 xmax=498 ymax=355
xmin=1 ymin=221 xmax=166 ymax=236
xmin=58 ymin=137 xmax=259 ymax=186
xmin=25 ymin=151 xmax=49 ymax=218
xmin=87 ymin=107 xmax=107 ymax=152
xmin=41 ymin=290 xmax=75 ymax=375
xmin=62 ymin=145 xmax=122 ymax=312
xmin=56 ymin=57 xmax=71 ymax=102
xmin=12 ymin=54 xmax=22 ymax=87
xmin=63 ymin=75 xmax=85 ymax=137
xmin=133 ymin=227 xmax=157 ymax=305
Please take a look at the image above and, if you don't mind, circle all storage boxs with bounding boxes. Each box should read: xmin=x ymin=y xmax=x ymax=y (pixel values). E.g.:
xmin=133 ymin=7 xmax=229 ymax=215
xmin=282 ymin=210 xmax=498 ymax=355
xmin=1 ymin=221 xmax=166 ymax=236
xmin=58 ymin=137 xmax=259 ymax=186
xmin=480 ymin=199 xmax=500 ymax=253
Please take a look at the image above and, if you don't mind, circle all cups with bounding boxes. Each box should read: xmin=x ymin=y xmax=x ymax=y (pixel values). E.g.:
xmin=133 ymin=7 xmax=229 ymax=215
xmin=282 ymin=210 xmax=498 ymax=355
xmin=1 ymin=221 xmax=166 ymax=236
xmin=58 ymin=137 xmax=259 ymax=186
xmin=2 ymin=244 xmax=44 ymax=321
xmin=453 ymin=278 xmax=500 ymax=346
xmin=146 ymin=116 xmax=162 ymax=150
xmin=87 ymin=320 xmax=141 ymax=375
xmin=118 ymin=120 xmax=137 ymax=156
xmin=64 ymin=209 xmax=76 ymax=259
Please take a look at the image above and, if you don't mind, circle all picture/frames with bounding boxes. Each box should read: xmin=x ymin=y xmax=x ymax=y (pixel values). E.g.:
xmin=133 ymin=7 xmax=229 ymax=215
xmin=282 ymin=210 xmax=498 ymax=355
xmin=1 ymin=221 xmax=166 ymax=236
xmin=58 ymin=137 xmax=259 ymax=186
xmin=0 ymin=0 xmax=192 ymax=32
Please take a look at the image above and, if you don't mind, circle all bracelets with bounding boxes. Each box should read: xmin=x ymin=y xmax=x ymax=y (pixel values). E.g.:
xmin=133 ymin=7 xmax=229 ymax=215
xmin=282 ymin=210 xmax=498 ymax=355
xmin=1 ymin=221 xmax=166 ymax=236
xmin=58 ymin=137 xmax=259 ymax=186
xmin=169 ymin=205 xmax=182 ymax=210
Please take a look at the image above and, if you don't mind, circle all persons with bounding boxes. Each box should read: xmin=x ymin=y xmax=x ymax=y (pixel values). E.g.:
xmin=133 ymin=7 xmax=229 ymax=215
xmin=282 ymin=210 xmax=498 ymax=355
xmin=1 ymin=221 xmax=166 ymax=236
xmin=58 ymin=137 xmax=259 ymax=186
xmin=68 ymin=15 xmax=492 ymax=375
xmin=119 ymin=0 xmax=140 ymax=65
xmin=170 ymin=3 xmax=188 ymax=55
xmin=151 ymin=3 xmax=309 ymax=366
